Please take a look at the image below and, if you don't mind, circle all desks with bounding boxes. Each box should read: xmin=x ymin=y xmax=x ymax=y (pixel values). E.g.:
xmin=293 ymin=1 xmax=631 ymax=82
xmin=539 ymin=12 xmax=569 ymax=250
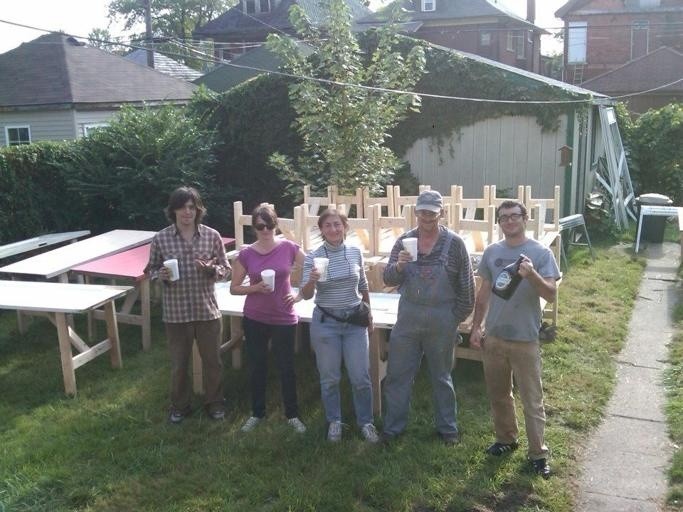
xmin=0 ymin=230 xmax=90 ymax=283
xmin=636 ymin=205 xmax=683 ymax=254
xmin=302 ymin=184 xmax=361 ymax=252
xmin=192 ymin=280 xmax=400 ymax=416
xmin=455 ymin=186 xmax=523 ymax=252
xmin=0 ymin=229 xmax=159 ymax=335
xmin=0 ymin=280 xmax=134 ymax=398
xmin=375 ymin=185 xmax=458 ymax=255
xmin=454 ymin=279 xmax=563 ymax=362
xmin=499 ymin=185 xmax=561 ymax=246
xmin=556 ymin=235 xmax=561 ymax=273
xmin=412 ymin=185 xmax=489 ymax=230
xmin=336 ymin=184 xmax=424 ymax=255
xmin=225 ymin=185 xmax=331 ymax=259
xmin=71 ymin=238 xmax=235 ymax=349
xmin=302 ymin=184 xmax=393 ymax=252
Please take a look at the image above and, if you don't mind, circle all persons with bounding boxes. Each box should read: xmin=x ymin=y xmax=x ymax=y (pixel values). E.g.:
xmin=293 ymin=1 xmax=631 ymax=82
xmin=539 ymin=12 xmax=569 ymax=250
xmin=380 ymin=190 xmax=476 ymax=450
xmin=300 ymin=208 xmax=379 ymax=444
xmin=231 ymin=204 xmax=311 ymax=435
xmin=471 ymin=199 xmax=561 ymax=480
xmin=144 ymin=187 xmax=233 ymax=424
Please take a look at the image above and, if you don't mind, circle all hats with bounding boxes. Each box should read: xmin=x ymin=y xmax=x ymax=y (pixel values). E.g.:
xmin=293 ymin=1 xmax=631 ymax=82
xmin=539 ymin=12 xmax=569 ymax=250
xmin=416 ymin=190 xmax=443 ymax=214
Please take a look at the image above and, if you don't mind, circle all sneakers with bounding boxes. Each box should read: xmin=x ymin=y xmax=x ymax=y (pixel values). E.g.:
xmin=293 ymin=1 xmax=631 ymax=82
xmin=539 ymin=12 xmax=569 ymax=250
xmin=441 ymin=433 xmax=459 ymax=447
xmin=240 ymin=416 xmax=265 ymax=433
xmin=288 ymin=417 xmax=306 ymax=434
xmin=381 ymin=432 xmax=395 ymax=445
xmin=170 ymin=404 xmax=192 ymax=424
xmin=211 ymin=411 xmax=224 ymax=419
xmin=532 ymin=458 xmax=551 ymax=480
xmin=328 ymin=421 xmax=351 ymax=442
xmin=360 ymin=423 xmax=379 ymax=444
xmin=486 ymin=439 xmax=518 ymax=456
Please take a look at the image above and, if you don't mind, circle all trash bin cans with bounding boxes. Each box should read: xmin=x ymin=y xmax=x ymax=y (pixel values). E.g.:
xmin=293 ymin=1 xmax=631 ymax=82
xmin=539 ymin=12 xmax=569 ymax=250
xmin=634 ymin=192 xmax=673 ymax=243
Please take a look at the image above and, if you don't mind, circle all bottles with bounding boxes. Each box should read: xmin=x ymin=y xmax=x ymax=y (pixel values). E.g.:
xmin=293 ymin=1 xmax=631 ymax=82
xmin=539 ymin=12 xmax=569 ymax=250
xmin=492 ymin=252 xmax=523 ymax=301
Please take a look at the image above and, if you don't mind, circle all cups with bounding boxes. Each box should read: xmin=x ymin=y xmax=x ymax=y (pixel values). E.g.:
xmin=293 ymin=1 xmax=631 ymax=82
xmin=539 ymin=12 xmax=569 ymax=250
xmin=402 ymin=237 xmax=418 ymax=263
xmin=260 ymin=268 xmax=276 ymax=292
xmin=163 ymin=258 xmax=181 ymax=282
xmin=313 ymin=257 xmax=330 ymax=282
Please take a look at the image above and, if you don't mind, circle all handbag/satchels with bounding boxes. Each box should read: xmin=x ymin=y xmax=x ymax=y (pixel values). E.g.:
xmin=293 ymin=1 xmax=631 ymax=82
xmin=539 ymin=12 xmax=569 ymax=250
xmin=347 ymin=301 xmax=370 ymax=326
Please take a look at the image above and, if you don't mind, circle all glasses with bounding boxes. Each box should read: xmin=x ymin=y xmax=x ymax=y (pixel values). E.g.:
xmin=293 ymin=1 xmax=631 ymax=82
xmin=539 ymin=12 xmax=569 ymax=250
xmin=496 ymin=214 xmax=523 ymax=222
xmin=253 ymin=223 xmax=276 ymax=231
xmin=328 ymin=262 xmax=350 ymax=281
